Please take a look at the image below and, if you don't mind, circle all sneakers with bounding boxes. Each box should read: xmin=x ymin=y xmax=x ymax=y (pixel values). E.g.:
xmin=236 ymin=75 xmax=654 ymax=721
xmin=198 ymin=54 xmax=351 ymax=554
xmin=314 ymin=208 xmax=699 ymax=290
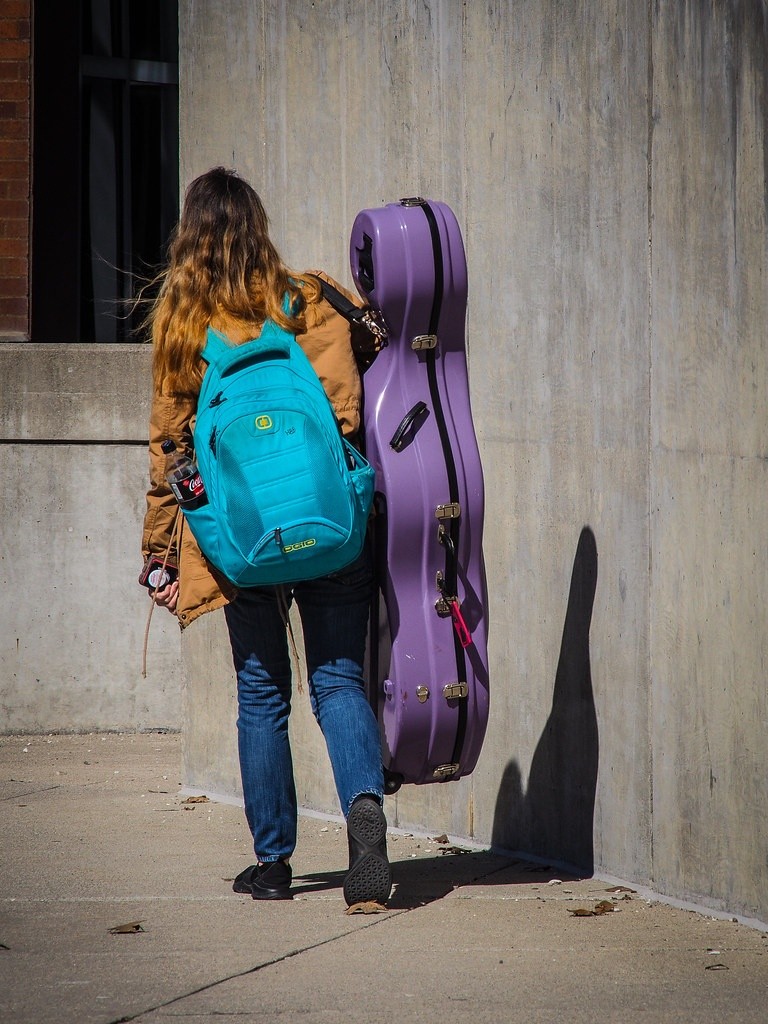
xmin=233 ymin=861 xmax=293 ymax=900
xmin=342 ymin=795 xmax=392 ymax=910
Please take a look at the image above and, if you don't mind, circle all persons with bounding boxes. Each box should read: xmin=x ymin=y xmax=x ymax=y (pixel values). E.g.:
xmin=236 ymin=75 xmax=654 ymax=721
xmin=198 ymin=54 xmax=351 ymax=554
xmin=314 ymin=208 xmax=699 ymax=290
xmin=133 ymin=165 xmax=405 ymax=913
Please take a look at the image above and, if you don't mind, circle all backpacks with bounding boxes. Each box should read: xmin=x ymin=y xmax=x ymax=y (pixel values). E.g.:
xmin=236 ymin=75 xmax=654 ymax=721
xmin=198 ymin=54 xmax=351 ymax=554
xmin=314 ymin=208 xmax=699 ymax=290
xmin=181 ymin=278 xmax=374 ymax=591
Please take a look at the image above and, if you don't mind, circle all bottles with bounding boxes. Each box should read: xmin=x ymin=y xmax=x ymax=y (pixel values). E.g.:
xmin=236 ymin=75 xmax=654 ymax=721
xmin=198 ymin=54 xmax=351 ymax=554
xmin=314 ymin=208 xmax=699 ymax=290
xmin=160 ymin=440 xmax=208 ymax=511
